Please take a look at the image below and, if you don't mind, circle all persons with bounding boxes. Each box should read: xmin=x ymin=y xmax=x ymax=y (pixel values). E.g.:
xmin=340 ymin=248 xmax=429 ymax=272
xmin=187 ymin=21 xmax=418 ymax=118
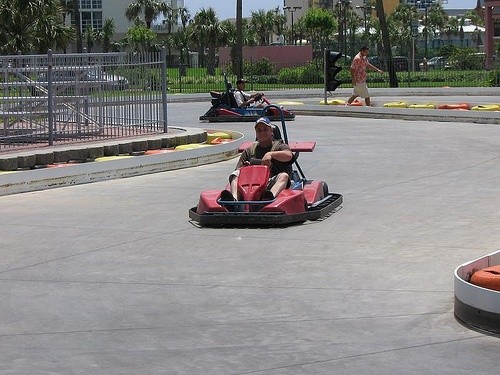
xmin=233 ymin=79 xmax=263 ymax=108
xmin=345 ymin=46 xmax=384 ymax=107
xmin=220 ymin=116 xmax=294 ymax=212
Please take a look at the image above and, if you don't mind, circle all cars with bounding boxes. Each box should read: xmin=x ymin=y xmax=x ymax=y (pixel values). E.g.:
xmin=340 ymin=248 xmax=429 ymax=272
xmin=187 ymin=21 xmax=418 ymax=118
xmin=26 ymin=65 xmax=129 ymax=96
xmin=368 ymin=56 xmax=410 ymax=72
xmin=419 ymin=52 xmax=486 ymax=70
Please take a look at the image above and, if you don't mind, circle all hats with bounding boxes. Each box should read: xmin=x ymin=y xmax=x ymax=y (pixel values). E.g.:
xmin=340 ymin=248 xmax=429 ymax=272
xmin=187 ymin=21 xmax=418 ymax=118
xmin=236 ymin=79 xmax=247 ymax=84
xmin=255 ymin=117 xmax=271 ymax=129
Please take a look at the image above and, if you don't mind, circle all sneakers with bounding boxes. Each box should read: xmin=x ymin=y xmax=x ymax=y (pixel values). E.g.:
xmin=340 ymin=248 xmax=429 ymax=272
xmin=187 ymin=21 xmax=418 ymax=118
xmin=221 ymin=190 xmax=239 ymax=214
xmin=260 ymin=190 xmax=275 ymax=205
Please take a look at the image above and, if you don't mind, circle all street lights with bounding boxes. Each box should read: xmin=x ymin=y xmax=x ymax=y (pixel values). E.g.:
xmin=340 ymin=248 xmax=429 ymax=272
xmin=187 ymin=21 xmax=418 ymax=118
xmin=337 ymin=1 xmax=352 ymax=64
xmin=283 ymin=6 xmax=302 ymax=45
xmin=355 ymin=5 xmax=376 ymax=41
